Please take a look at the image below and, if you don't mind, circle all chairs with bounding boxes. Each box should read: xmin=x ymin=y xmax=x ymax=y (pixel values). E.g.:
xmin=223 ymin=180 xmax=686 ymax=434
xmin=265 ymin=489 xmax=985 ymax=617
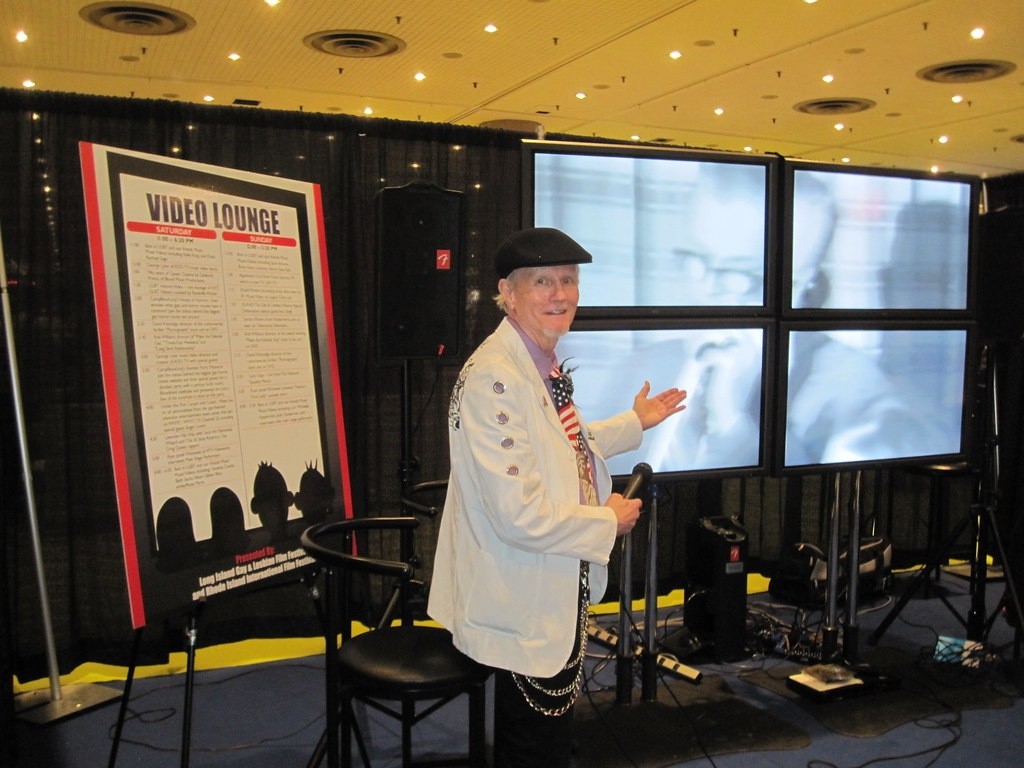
xmin=303 ymin=516 xmax=497 ymax=768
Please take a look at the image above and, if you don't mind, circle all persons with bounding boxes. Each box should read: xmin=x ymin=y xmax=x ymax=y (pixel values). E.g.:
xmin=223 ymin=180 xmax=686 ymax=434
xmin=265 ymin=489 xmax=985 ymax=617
xmin=426 ymin=228 xmax=688 ymax=768
xmin=599 ymin=167 xmax=929 ymax=474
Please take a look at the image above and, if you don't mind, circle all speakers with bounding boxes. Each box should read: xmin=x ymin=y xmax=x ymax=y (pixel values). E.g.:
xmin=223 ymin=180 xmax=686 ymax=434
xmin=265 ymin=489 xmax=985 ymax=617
xmin=370 ymin=178 xmax=471 ymax=360
xmin=683 ymin=510 xmax=749 ymax=655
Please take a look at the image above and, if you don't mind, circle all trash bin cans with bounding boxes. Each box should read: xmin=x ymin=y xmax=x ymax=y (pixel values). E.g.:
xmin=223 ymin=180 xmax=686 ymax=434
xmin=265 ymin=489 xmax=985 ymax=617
xmin=686 ymin=514 xmax=748 ymax=655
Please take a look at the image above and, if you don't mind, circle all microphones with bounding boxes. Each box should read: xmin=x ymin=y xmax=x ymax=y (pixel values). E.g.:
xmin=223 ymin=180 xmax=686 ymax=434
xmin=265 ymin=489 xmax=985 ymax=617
xmin=622 ymin=462 xmax=653 ymax=501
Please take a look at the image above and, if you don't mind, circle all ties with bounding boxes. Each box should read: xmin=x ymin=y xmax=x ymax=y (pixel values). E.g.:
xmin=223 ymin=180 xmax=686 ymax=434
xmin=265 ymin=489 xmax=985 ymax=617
xmin=548 ymin=368 xmax=597 ymax=507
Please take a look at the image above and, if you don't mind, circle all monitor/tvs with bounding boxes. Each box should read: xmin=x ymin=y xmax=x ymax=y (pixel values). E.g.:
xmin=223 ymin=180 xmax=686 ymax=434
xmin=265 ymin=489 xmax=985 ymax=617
xmin=520 ymin=138 xmax=778 ymax=316
xmin=771 ymin=320 xmax=979 ymax=477
xmin=551 ymin=317 xmax=776 ymax=487
xmin=780 ymin=164 xmax=981 ymax=322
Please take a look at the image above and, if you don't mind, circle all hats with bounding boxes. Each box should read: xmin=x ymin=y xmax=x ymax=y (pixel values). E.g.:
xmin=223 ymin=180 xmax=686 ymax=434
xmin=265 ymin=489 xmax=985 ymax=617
xmin=495 ymin=228 xmax=592 ymax=279
